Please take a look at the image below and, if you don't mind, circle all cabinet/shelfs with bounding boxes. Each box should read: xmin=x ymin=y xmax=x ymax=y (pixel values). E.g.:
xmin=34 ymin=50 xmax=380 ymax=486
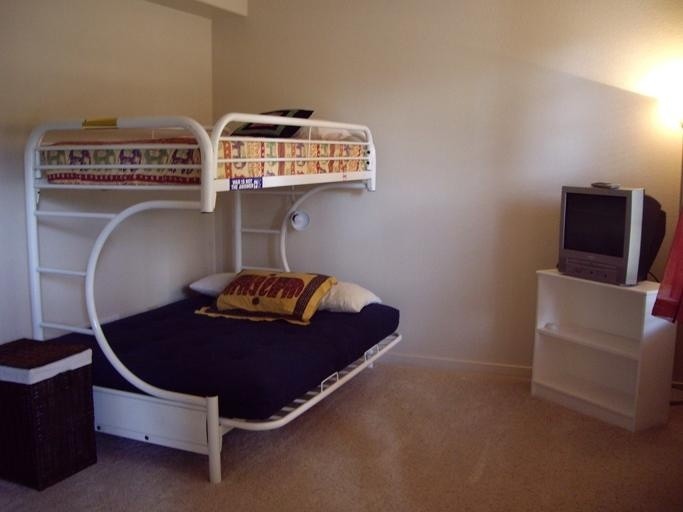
xmin=531 ymin=269 xmax=678 ymax=432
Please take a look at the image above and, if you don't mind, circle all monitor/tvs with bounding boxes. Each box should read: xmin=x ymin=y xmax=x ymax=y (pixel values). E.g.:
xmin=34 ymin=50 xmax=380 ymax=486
xmin=559 ymin=187 xmax=665 ymax=286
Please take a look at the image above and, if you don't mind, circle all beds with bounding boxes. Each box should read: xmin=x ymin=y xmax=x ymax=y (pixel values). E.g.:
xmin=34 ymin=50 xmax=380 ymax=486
xmin=24 ymin=112 xmax=402 ymax=484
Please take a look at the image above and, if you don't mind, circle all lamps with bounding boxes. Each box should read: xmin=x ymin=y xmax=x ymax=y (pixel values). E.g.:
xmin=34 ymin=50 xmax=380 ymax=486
xmin=289 ymin=211 xmax=310 ymax=232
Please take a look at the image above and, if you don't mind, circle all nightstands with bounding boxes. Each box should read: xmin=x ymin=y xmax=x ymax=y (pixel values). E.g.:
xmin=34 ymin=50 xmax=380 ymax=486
xmin=0 ymin=338 xmax=97 ymax=491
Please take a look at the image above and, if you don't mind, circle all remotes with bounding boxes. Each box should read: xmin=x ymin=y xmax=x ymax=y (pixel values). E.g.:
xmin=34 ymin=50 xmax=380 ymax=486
xmin=592 ymin=182 xmax=620 ymax=189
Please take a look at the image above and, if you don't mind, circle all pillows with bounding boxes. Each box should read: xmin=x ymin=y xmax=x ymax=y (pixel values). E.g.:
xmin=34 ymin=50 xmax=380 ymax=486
xmin=194 ymin=268 xmax=337 ymax=325
xmin=189 ymin=272 xmax=237 ymax=297
xmin=231 ymin=109 xmax=314 ymax=138
xmin=292 ymin=123 xmax=352 ymax=140
xmin=319 ymin=281 xmax=382 ymax=314
